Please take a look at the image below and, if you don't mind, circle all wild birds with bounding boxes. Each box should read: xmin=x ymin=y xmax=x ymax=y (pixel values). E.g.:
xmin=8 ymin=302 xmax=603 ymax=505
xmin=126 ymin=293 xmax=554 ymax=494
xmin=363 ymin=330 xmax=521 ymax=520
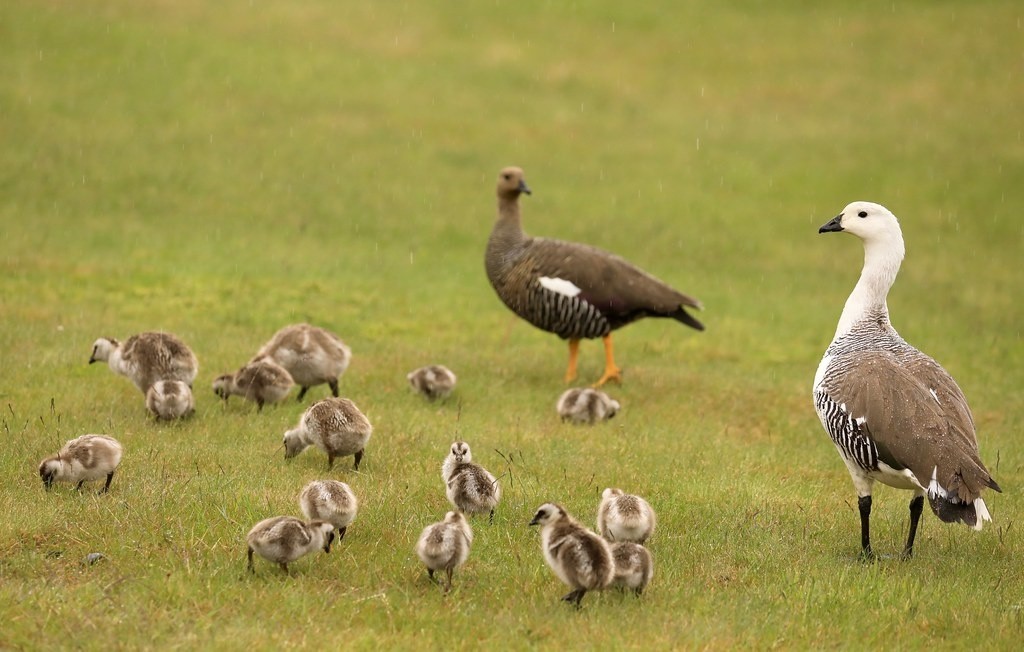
xmin=243 ymin=478 xmax=359 ymax=576
xmin=556 ymin=387 xmax=622 ymax=426
xmin=405 ymin=363 xmax=458 ymax=402
xmin=37 ymin=329 xmax=201 ymax=497
xmin=808 ymin=199 xmax=1007 ymax=571
xmin=413 ymin=440 xmax=503 ymax=591
xmin=483 ymin=164 xmax=706 ymax=390
xmin=525 ymin=486 xmax=658 ymax=613
xmin=210 ymin=320 xmax=373 ymax=473
